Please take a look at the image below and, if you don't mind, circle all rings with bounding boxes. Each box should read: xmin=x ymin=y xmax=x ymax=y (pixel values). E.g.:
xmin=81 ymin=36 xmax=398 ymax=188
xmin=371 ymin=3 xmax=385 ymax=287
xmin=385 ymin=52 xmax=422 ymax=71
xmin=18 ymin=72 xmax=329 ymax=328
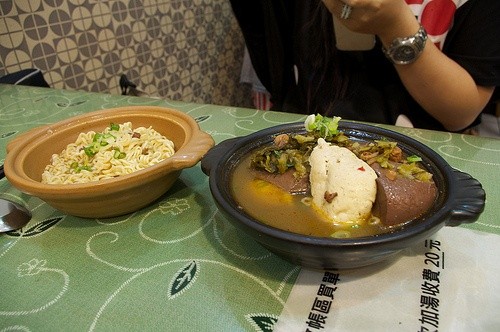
xmin=340 ymin=5 xmax=352 ymax=20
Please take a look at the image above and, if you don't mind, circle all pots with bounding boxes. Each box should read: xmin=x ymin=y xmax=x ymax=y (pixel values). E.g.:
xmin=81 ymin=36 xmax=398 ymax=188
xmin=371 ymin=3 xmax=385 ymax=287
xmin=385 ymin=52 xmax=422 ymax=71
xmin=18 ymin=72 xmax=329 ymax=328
xmin=4 ymin=106 xmax=216 ymax=219
xmin=200 ymin=121 xmax=486 ymax=275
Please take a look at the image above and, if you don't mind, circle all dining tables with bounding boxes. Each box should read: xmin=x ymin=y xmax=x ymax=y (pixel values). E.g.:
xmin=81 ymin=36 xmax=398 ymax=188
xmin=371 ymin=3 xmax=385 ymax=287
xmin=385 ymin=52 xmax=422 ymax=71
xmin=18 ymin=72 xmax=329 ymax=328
xmin=0 ymin=83 xmax=499 ymax=331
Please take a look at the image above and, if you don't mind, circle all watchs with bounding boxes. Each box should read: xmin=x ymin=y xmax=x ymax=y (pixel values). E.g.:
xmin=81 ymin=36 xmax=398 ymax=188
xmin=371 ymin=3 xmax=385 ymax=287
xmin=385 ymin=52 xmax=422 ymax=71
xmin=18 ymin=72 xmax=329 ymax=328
xmin=382 ymin=23 xmax=428 ymax=65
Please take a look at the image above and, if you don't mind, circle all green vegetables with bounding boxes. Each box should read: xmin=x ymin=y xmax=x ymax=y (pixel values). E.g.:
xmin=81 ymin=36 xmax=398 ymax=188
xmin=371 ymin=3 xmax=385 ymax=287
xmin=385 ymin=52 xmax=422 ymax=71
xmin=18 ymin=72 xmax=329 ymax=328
xmin=307 ymin=114 xmax=341 ymax=140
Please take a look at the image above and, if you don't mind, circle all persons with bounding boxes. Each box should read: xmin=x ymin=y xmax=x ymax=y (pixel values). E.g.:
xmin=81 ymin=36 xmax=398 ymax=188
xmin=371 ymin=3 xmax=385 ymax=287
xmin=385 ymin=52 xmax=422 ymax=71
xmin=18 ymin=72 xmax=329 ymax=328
xmin=230 ymin=0 xmax=500 ymax=134
